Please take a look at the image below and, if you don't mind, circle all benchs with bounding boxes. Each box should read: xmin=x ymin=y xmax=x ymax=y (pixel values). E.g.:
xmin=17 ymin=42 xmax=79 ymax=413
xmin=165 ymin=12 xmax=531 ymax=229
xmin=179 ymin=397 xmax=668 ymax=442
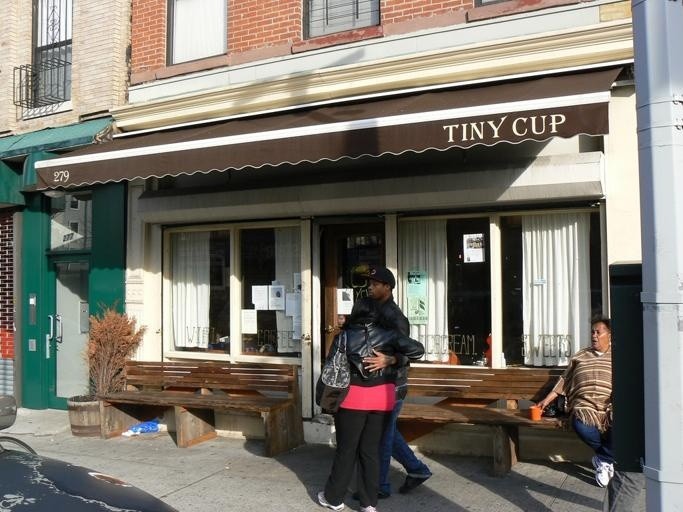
xmin=93 ymin=359 xmax=304 ymax=455
xmin=394 ymin=366 xmax=574 ymax=476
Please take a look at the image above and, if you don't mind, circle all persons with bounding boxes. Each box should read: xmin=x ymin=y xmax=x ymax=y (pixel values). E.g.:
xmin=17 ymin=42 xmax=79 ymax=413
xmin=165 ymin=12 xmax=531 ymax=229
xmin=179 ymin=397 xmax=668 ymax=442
xmin=537 ymin=317 xmax=623 ymax=488
xmin=350 ymin=266 xmax=433 ymax=502
xmin=317 ymin=300 xmax=424 ymax=512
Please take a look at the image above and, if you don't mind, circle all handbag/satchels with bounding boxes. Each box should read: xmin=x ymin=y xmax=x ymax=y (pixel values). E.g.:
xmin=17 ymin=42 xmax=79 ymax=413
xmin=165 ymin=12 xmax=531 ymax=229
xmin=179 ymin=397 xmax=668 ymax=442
xmin=316 ymin=331 xmax=351 ymax=413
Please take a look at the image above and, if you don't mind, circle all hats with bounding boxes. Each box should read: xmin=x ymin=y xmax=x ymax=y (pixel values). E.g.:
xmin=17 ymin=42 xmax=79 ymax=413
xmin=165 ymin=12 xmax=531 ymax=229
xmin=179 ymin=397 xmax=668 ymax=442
xmin=360 ymin=267 xmax=395 ymax=287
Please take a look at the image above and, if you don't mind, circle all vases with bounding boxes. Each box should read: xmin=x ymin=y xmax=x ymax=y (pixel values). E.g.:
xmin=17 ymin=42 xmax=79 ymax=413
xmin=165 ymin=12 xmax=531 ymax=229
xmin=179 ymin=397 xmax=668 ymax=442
xmin=68 ymin=395 xmax=102 ymax=437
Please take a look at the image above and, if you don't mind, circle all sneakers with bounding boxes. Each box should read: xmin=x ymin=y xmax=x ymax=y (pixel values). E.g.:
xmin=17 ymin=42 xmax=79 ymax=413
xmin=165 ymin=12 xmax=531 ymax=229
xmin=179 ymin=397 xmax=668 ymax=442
xmin=398 ymin=470 xmax=432 ymax=494
xmin=352 ymin=487 xmax=391 ymax=500
xmin=318 ymin=491 xmax=344 ymax=511
xmin=591 ymin=454 xmax=614 ymax=487
xmin=359 ymin=505 xmax=379 ymax=512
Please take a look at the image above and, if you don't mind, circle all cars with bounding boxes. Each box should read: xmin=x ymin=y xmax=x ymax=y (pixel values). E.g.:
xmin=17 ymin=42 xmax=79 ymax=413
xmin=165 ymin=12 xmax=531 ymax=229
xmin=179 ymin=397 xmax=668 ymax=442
xmin=0 ymin=395 xmax=177 ymax=512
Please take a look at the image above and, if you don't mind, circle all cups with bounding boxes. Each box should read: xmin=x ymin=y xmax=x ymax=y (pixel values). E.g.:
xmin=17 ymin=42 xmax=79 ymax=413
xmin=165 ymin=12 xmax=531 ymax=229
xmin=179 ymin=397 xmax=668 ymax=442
xmin=529 ymin=405 xmax=542 ymax=421
